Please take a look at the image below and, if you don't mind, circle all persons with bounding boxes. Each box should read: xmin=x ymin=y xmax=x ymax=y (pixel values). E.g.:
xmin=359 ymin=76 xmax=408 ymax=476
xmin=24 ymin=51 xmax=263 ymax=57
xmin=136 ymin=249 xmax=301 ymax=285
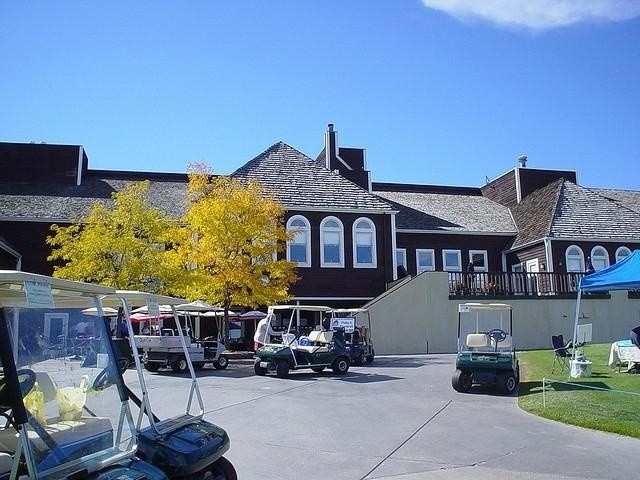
xmin=254 ymin=311 xmax=285 ymax=351
xmin=121 ymin=319 xmax=132 ymax=338
xmin=75 ymin=318 xmax=98 ymax=337
xmin=630 ymin=326 xmax=640 ymax=349
xmin=107 ymin=318 xmax=116 ymax=337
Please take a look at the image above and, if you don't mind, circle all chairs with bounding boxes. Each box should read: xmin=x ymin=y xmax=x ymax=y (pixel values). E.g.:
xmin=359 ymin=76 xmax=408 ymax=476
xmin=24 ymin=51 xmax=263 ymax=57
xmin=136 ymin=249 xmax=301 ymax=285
xmin=162 ymin=328 xmax=187 ymax=337
xmin=466 ymin=333 xmax=512 ymax=351
xmin=551 ymin=334 xmax=574 ymax=373
xmin=308 ymin=330 xmax=334 ymax=343
xmin=0 ymin=370 xmax=113 ymax=472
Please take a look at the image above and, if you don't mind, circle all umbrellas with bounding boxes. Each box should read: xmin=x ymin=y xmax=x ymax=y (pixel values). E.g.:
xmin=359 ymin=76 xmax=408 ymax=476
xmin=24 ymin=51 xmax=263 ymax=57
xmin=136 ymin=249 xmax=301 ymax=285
xmin=81 ymin=307 xmax=118 ymax=317
xmin=130 ymin=300 xmax=267 ymax=336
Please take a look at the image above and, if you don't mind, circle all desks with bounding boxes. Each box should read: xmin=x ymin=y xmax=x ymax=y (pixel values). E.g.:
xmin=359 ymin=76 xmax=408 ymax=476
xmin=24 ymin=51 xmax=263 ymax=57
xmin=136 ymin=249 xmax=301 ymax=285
xmin=570 ymin=360 xmax=592 ymax=378
xmin=608 ymin=341 xmax=640 ymax=373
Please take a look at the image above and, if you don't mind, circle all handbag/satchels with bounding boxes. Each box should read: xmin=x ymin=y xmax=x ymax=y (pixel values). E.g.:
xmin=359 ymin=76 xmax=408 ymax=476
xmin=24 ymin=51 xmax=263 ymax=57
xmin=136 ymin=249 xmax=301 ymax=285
xmin=23 ymin=381 xmax=47 ymax=431
xmin=56 ymin=375 xmax=89 ymax=421
